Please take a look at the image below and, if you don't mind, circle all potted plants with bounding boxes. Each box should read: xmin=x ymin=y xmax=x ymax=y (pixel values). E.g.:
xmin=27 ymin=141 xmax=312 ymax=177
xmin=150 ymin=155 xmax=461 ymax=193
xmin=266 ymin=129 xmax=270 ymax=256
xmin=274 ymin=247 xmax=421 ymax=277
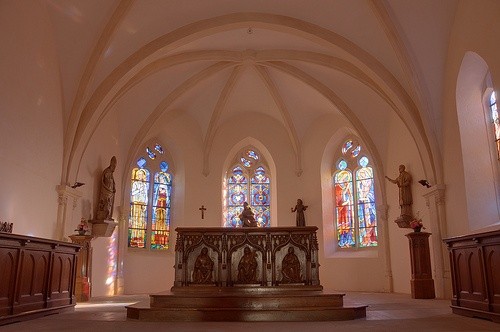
xmin=74 ymin=224 xmax=86 ymax=235
xmin=410 ymin=219 xmax=423 ymax=232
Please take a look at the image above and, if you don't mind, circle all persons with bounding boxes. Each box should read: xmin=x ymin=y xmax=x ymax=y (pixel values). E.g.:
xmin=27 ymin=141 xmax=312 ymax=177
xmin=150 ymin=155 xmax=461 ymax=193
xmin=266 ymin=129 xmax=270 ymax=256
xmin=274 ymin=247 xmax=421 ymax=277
xmin=385 ymin=165 xmax=414 ymax=217
xmin=281 ymin=247 xmax=304 ymax=282
xmin=193 ymin=248 xmax=214 ymax=282
xmin=94 ymin=155 xmax=118 ymax=220
xmin=239 ymin=202 xmax=258 ymax=227
xmin=291 ymin=198 xmax=310 ymax=227
xmin=237 ymin=246 xmax=259 ymax=283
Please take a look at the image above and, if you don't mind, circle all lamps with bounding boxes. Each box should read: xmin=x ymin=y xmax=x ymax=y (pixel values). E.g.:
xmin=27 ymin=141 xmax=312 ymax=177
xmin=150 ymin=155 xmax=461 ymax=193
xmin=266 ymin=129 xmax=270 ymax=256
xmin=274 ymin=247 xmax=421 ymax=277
xmin=71 ymin=182 xmax=84 ymax=189
xmin=418 ymin=180 xmax=431 ymax=189
